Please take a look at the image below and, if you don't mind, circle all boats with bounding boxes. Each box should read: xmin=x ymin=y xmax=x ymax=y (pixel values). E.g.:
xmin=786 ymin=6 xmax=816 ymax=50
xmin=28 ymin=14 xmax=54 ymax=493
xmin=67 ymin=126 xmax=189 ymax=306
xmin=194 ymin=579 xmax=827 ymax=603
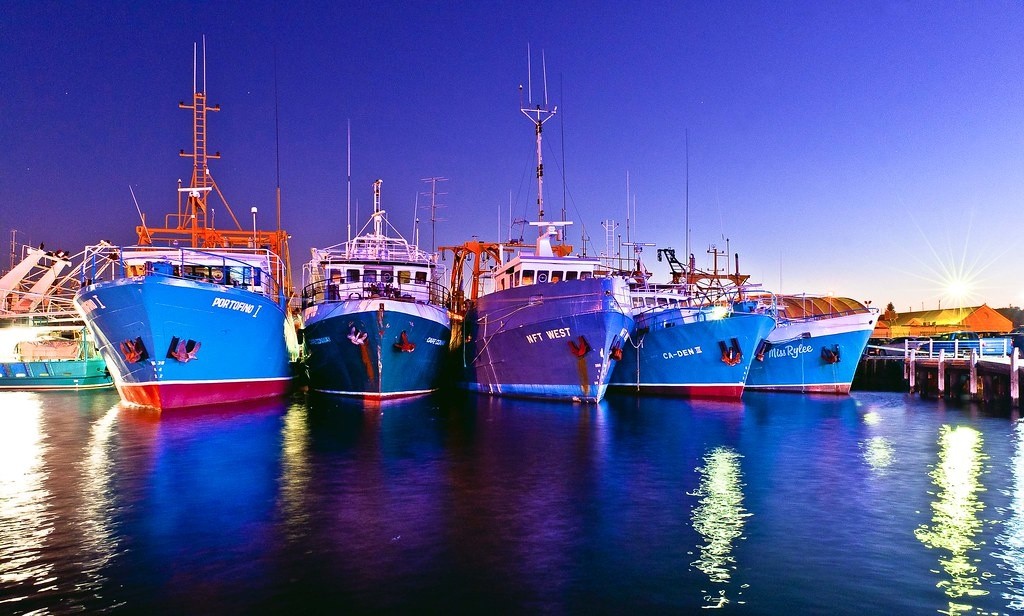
xmin=441 ymin=38 xmax=641 ymax=409
xmin=593 ymin=125 xmax=780 ymax=403
xmin=736 ymin=291 xmax=881 ymax=399
xmin=1 ymin=227 xmax=114 ymax=391
xmin=297 ymin=116 xmax=467 ymax=402
xmin=72 ymin=31 xmax=304 ymax=414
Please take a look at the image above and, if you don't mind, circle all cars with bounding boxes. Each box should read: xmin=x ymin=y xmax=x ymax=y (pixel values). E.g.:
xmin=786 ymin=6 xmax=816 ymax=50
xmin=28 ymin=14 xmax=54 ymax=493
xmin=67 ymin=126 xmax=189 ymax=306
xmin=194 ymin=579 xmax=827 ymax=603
xmin=861 ymin=337 xmax=896 ymax=356
xmin=876 ymin=336 xmax=933 ymax=357
xmin=916 ymin=331 xmax=985 ymax=356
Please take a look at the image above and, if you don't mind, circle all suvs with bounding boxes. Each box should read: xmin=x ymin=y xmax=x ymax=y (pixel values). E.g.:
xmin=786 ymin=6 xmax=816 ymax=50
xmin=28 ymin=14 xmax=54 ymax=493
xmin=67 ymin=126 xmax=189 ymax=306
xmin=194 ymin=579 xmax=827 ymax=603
xmin=1004 ymin=325 xmax=1024 ymax=360
